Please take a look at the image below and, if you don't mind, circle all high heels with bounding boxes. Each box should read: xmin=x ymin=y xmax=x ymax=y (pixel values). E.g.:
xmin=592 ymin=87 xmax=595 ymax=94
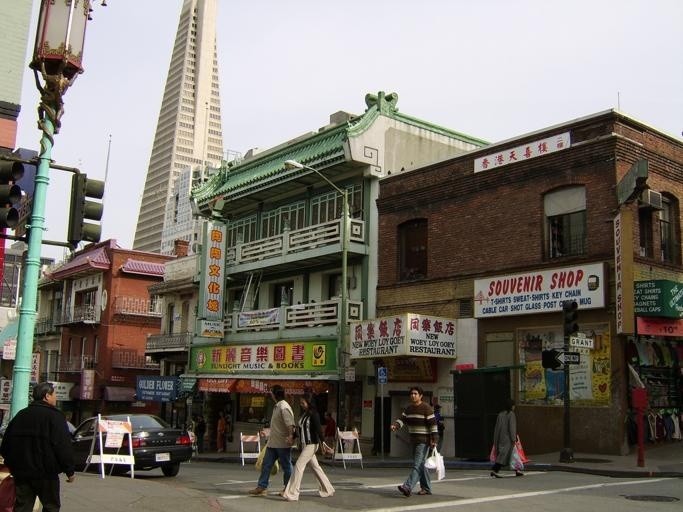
xmin=490 ymin=472 xmax=503 ymax=478
xmin=516 ymin=471 xmax=523 ymax=476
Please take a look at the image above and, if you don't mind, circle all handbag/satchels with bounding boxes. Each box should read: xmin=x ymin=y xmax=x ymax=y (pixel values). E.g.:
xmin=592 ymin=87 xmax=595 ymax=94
xmin=255 ymin=443 xmax=279 ymax=475
xmin=424 ymin=445 xmax=446 ymax=481
xmin=0 ymin=474 xmax=16 ymax=512
xmin=510 ymin=445 xmax=523 ymax=470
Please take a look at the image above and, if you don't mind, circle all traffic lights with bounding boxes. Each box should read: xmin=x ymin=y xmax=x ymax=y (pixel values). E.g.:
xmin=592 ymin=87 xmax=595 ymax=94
xmin=68 ymin=174 xmax=105 ymax=243
xmin=542 ymin=352 xmax=557 ymax=367
xmin=0 ymin=155 xmax=24 ymax=228
xmin=564 ymin=301 xmax=578 ymax=337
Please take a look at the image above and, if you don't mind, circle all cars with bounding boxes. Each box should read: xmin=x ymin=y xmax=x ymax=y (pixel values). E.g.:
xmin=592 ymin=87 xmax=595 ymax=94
xmin=66 ymin=413 xmax=193 ymax=477
xmin=67 ymin=420 xmax=76 ymax=435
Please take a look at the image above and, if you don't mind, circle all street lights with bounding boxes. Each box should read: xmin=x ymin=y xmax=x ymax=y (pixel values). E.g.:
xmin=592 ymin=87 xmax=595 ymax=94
xmin=283 ymin=159 xmax=350 ymax=452
xmin=9 ymin=0 xmax=106 ymax=423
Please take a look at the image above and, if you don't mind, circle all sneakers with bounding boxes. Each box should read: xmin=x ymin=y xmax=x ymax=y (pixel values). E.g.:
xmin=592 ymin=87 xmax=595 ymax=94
xmin=248 ymin=486 xmax=267 ymax=496
xmin=418 ymin=490 xmax=427 ymax=495
xmin=398 ymin=485 xmax=410 ymax=497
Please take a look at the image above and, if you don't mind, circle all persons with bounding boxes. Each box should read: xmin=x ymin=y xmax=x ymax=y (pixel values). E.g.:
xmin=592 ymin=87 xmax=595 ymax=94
xmin=428 ymin=402 xmax=435 ymax=412
xmin=433 ymin=404 xmax=445 ymax=452
xmin=488 ymin=398 xmax=524 ymax=477
xmin=0 ymin=382 xmax=76 ymax=512
xmin=217 ymin=412 xmax=225 ymax=453
xmin=323 ymin=410 xmax=335 ymax=444
xmin=247 ymin=384 xmax=296 ymax=496
xmin=276 ymin=393 xmax=335 ymax=500
xmin=196 ymin=415 xmax=207 ymax=453
xmin=390 ymin=385 xmax=439 ymax=496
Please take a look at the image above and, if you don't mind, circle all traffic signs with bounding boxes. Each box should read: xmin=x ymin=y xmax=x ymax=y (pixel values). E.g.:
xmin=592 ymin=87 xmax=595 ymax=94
xmin=555 ymin=352 xmax=580 ymax=365
xmin=570 ymin=336 xmax=594 ymax=349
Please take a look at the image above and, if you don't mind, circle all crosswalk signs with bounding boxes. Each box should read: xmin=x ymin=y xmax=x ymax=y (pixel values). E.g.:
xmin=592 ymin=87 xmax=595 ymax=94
xmin=376 ymin=366 xmax=387 ymax=384
